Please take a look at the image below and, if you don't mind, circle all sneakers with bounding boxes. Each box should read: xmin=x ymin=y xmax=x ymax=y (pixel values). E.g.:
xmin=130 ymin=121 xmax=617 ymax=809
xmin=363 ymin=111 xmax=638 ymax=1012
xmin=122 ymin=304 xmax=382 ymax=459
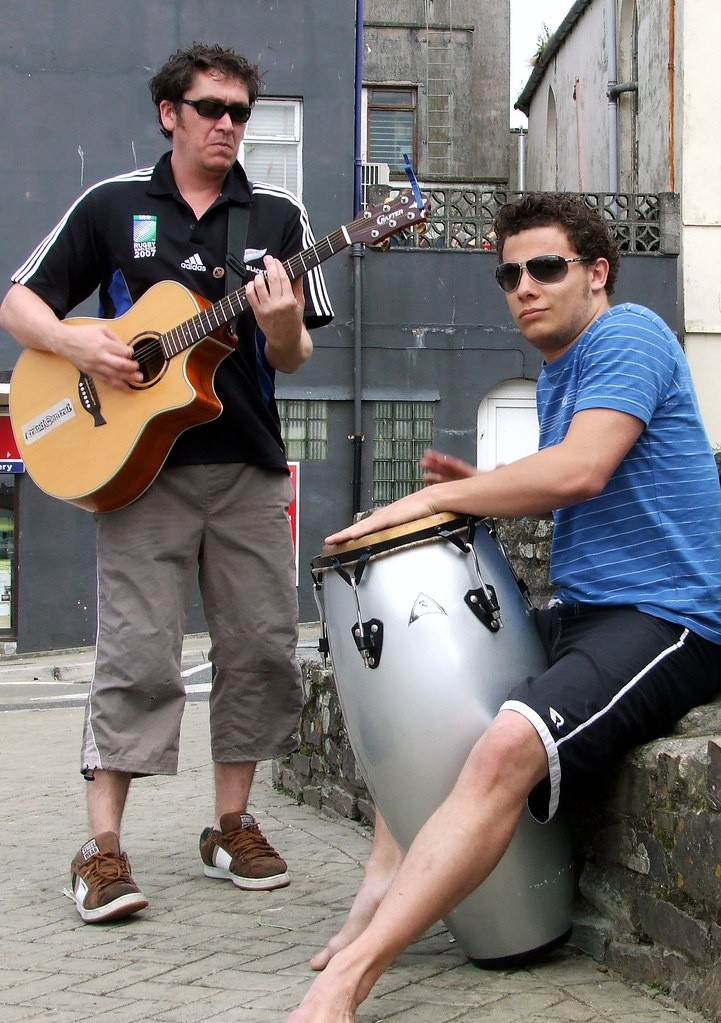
xmin=71 ymin=831 xmax=149 ymax=922
xmin=199 ymin=811 xmax=291 ymax=891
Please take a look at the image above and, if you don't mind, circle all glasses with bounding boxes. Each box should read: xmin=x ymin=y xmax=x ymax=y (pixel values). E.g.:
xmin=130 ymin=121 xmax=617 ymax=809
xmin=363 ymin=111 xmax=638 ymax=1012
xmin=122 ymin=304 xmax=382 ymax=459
xmin=176 ymin=99 xmax=252 ymax=123
xmin=495 ymin=254 xmax=595 ymax=293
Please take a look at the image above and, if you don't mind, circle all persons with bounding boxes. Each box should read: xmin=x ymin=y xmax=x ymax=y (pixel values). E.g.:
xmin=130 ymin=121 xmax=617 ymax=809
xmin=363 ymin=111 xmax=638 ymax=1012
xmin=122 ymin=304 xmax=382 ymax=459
xmin=0 ymin=45 xmax=314 ymax=925
xmin=281 ymin=192 xmax=720 ymax=1022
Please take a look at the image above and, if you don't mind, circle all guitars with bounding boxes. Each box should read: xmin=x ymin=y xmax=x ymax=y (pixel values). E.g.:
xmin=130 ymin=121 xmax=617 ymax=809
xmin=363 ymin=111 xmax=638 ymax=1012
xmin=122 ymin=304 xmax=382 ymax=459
xmin=7 ymin=152 xmax=433 ymax=514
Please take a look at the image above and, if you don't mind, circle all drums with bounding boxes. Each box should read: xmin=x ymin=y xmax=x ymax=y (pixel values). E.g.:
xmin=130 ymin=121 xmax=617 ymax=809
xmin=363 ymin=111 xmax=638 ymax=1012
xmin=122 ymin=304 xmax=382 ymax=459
xmin=308 ymin=510 xmax=575 ymax=968
